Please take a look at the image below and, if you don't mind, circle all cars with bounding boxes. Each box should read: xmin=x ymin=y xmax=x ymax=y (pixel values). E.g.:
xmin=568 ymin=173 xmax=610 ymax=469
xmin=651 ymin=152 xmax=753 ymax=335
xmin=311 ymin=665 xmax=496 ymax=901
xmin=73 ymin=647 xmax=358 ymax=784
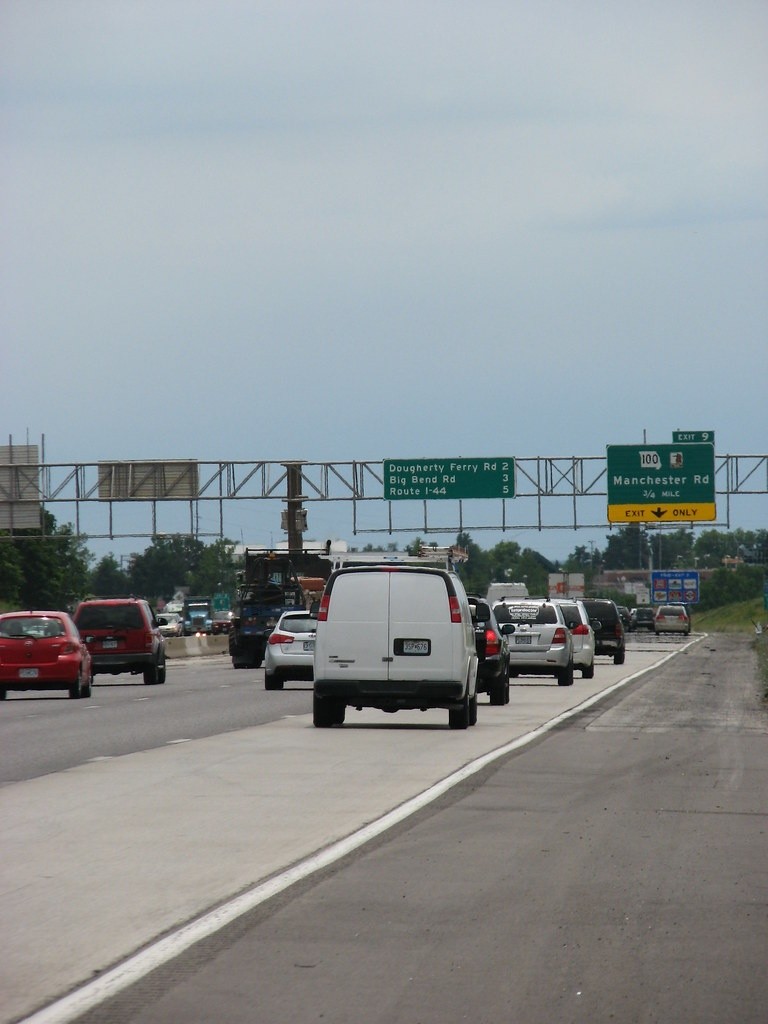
xmin=654 ymin=605 xmax=690 ymax=636
xmin=264 ymin=609 xmax=316 ymax=690
xmin=615 ymin=604 xmax=631 ymax=633
xmin=629 ymin=607 xmax=655 ymax=631
xmin=154 ymin=613 xmax=182 ymax=637
xmin=210 ymin=610 xmax=236 ymax=634
xmin=0 ymin=610 xmax=95 ymax=701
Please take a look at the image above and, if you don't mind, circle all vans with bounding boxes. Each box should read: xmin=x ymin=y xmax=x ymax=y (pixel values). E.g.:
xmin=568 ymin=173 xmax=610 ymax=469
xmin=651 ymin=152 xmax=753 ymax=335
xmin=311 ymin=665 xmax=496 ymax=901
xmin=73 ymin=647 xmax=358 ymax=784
xmin=310 ymin=555 xmax=491 ymax=729
xmin=485 ymin=582 xmax=530 ymax=607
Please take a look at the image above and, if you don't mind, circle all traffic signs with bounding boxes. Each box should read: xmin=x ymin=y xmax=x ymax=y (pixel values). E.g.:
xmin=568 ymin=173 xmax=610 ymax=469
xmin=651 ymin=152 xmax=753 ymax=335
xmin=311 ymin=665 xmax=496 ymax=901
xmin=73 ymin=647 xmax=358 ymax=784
xmin=605 ymin=430 xmax=718 ymax=523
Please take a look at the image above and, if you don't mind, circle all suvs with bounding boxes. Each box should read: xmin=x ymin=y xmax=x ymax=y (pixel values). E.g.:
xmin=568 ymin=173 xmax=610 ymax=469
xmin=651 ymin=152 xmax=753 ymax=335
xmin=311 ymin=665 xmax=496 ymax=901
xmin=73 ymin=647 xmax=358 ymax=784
xmin=547 ymin=596 xmax=602 ymax=678
xmin=465 ymin=590 xmax=516 ymax=706
xmin=491 ymin=596 xmax=579 ymax=687
xmin=566 ymin=597 xmax=627 ymax=664
xmin=72 ymin=594 xmax=169 ymax=686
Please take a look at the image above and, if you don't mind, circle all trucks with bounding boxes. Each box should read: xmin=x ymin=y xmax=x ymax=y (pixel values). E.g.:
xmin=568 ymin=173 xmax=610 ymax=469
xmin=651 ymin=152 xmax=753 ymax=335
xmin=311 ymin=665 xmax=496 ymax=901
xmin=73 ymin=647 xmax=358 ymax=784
xmin=183 ymin=595 xmax=214 ymax=636
xmin=228 ymin=539 xmax=339 ymax=670
